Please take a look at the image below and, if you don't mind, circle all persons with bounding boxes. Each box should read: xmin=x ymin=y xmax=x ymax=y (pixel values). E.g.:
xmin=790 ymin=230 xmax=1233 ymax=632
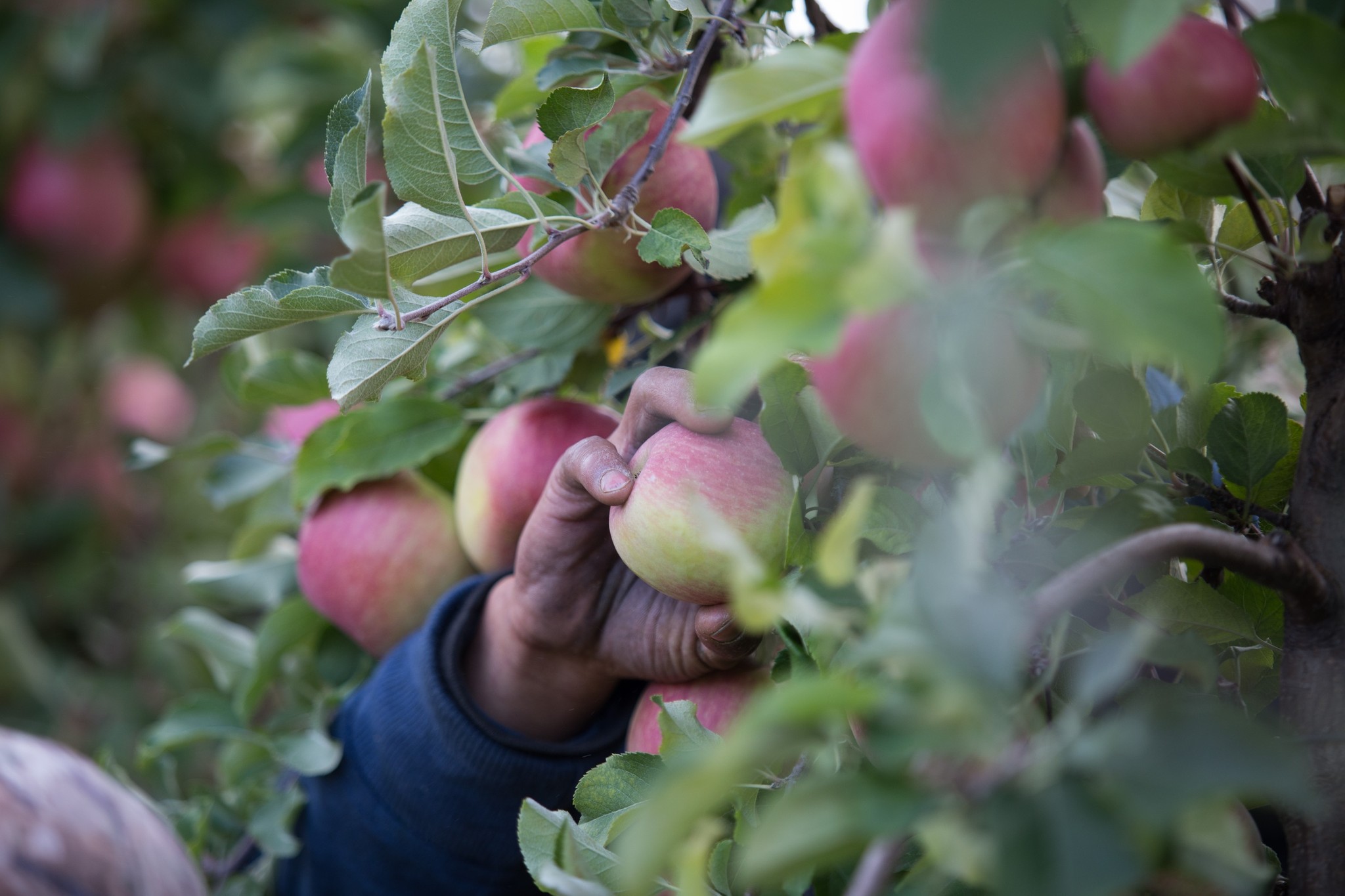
xmin=1 ymin=364 xmax=775 ymax=896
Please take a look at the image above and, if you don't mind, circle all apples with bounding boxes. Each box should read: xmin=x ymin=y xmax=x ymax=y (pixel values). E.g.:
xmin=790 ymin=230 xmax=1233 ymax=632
xmin=624 ymin=677 xmax=753 ymax=762
xmin=497 ymin=90 xmax=719 ymax=307
xmin=1 ymin=110 xmax=413 ymax=535
xmin=292 ymin=465 xmax=467 ymax=658
xmin=607 ymin=418 xmax=796 ymax=608
xmin=450 ymin=392 xmax=619 ymax=573
xmin=800 ymin=0 xmax=1257 ymax=487
